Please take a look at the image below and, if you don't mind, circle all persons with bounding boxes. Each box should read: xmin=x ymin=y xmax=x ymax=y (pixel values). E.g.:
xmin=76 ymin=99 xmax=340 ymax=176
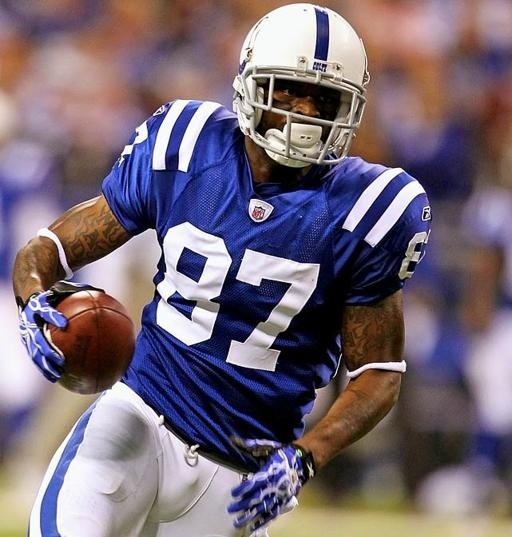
xmin=13 ymin=3 xmax=429 ymax=536
xmin=2 ymin=2 xmax=512 ymax=517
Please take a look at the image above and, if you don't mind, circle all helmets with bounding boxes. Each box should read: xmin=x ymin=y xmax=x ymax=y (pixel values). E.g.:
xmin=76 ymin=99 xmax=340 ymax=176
xmin=231 ymin=2 xmax=371 ymax=169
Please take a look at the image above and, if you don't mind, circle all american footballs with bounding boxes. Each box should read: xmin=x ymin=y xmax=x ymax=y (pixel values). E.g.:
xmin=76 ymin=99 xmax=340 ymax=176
xmin=42 ymin=289 xmax=137 ymax=395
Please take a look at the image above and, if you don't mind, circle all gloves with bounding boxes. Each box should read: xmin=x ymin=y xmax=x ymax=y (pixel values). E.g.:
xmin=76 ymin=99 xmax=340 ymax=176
xmin=228 ymin=436 xmax=316 ymax=531
xmin=15 ymin=280 xmax=105 ymax=383
